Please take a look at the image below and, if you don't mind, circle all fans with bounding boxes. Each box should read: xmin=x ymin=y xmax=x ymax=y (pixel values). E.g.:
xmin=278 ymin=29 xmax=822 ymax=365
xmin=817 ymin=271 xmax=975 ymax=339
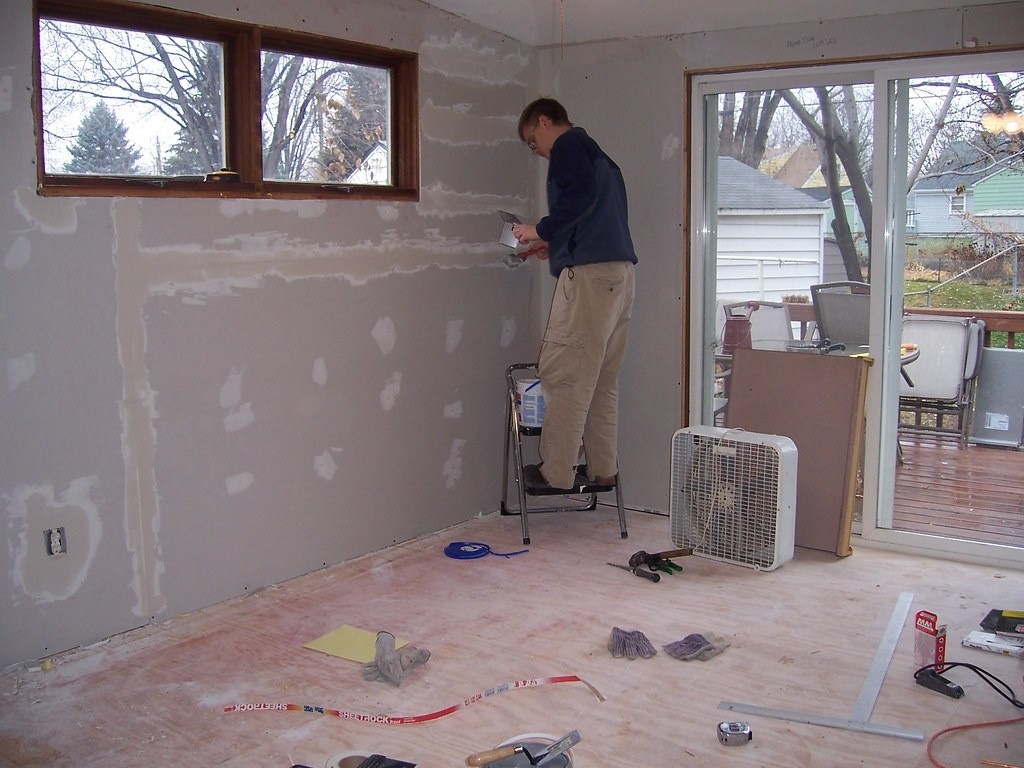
xmin=669 ymin=425 xmax=799 ymax=572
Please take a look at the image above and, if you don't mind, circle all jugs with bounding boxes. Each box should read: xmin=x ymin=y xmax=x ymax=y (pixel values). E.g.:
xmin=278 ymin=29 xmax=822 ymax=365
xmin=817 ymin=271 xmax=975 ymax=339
xmin=720 ymin=301 xmax=759 ymax=355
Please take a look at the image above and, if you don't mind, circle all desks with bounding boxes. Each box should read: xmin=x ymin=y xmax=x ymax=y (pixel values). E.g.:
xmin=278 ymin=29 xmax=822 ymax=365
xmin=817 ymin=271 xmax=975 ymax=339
xmin=715 ymin=339 xmax=919 ymax=464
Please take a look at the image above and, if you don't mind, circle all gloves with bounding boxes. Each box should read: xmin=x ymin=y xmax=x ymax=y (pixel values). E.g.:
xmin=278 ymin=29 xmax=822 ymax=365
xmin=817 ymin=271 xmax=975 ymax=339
xmin=360 ymin=630 xmax=430 ymax=686
xmin=608 ymin=628 xmax=656 ymax=662
xmin=664 ymin=634 xmax=730 ymax=661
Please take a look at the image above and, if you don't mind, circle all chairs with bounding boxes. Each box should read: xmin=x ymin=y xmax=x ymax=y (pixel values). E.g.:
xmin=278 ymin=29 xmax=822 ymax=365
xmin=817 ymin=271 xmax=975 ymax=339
xmin=723 ymin=301 xmax=794 ymax=341
xmin=501 ymin=363 xmax=628 ymax=545
xmin=810 ymin=281 xmax=904 ymax=343
xmin=899 ymin=312 xmax=984 ymax=450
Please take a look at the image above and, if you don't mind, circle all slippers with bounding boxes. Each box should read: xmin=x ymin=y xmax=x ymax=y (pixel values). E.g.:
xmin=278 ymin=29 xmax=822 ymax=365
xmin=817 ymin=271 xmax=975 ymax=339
xmin=337 ymin=754 xmax=417 ymax=768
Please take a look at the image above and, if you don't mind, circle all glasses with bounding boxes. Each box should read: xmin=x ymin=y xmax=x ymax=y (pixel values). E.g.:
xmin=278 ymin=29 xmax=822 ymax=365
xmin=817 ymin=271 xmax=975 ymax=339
xmin=527 ymin=118 xmax=550 ymax=151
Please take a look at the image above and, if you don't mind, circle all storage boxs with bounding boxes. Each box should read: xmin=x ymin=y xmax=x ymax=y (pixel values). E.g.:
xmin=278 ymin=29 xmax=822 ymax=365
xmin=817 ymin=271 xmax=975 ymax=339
xmin=914 ymin=611 xmax=947 ymax=671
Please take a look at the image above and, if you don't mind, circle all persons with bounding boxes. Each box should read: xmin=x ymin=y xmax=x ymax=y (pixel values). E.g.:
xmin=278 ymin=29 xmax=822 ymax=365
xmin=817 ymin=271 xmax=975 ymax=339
xmin=513 ymin=97 xmax=638 ymax=489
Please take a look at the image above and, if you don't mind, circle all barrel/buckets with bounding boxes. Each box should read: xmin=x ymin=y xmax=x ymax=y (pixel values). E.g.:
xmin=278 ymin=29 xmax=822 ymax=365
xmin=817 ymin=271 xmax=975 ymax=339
xmin=480 ymin=732 xmax=574 ymax=768
xmin=516 ymin=379 xmax=545 ymax=427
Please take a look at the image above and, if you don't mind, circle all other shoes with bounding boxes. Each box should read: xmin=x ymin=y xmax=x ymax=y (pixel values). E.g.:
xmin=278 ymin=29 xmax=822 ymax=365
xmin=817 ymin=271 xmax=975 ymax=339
xmin=524 ymin=464 xmax=555 ymax=489
xmin=576 ymin=465 xmax=618 ymax=487
